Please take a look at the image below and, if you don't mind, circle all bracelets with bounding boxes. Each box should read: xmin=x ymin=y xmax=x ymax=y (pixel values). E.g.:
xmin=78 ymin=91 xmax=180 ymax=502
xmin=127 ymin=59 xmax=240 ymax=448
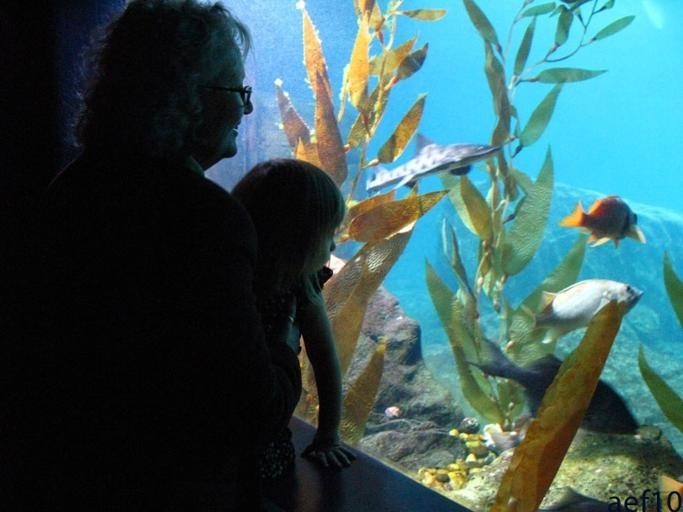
xmin=286 ymin=314 xmax=299 ymax=327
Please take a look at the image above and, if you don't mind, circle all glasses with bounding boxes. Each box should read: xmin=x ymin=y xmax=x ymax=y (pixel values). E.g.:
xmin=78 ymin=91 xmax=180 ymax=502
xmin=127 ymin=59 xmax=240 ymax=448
xmin=201 ymin=86 xmax=251 ymax=102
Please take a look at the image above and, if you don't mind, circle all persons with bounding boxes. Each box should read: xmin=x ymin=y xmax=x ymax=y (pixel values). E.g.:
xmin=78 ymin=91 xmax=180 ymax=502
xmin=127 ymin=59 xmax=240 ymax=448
xmin=18 ymin=0 xmax=304 ymax=512
xmin=216 ymin=158 xmax=356 ymax=512
